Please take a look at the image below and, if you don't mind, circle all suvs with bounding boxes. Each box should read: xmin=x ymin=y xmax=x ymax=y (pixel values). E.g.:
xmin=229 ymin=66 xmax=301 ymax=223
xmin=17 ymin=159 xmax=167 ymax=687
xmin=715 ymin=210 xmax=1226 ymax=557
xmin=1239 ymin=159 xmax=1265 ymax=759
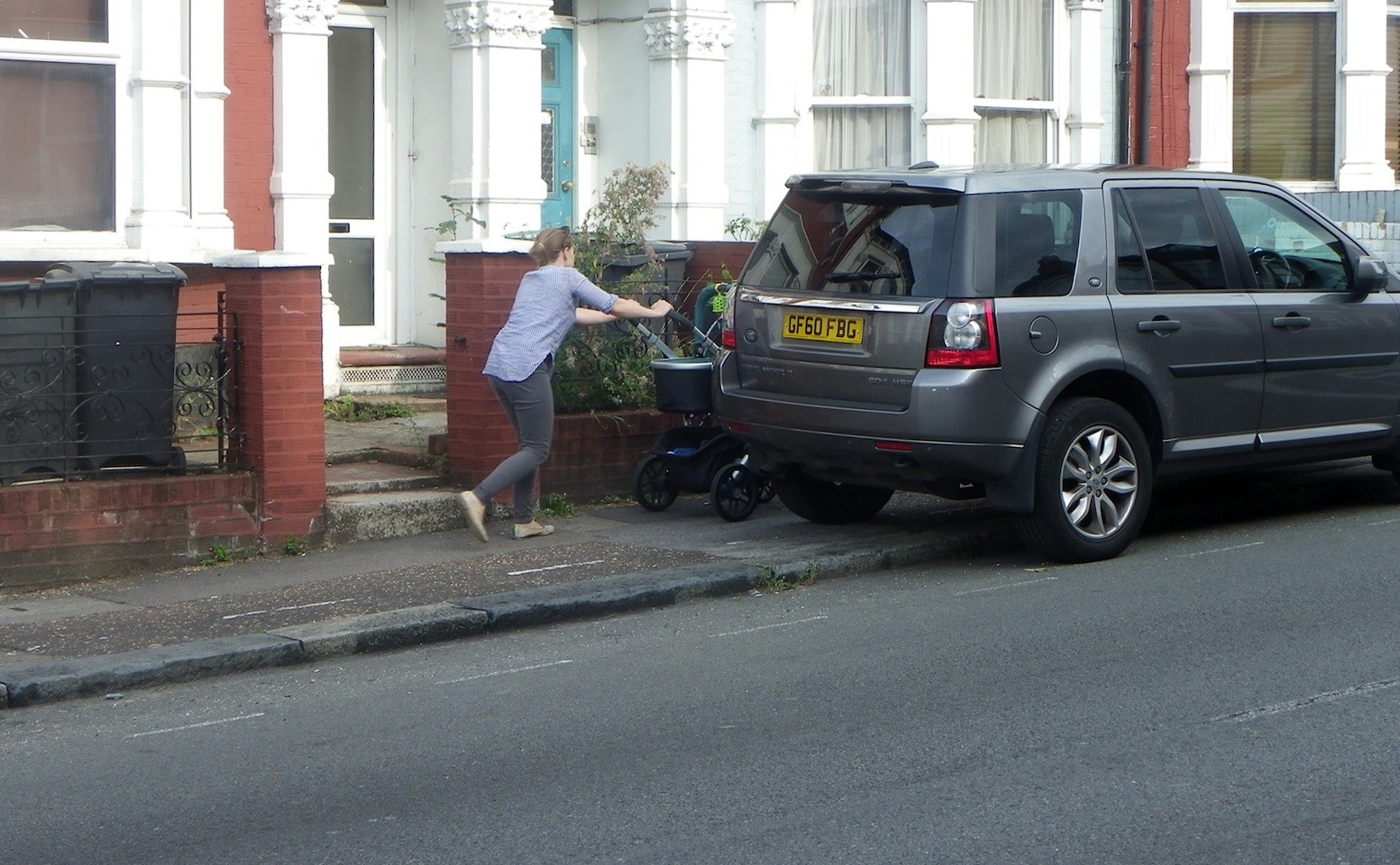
xmin=711 ymin=161 xmax=1400 ymax=562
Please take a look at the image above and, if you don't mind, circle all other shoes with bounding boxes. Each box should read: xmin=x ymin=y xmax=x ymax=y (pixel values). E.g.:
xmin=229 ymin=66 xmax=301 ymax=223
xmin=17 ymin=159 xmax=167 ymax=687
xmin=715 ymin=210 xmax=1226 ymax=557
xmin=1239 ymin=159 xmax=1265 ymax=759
xmin=455 ymin=490 xmax=488 ymax=542
xmin=511 ymin=518 xmax=554 ymax=541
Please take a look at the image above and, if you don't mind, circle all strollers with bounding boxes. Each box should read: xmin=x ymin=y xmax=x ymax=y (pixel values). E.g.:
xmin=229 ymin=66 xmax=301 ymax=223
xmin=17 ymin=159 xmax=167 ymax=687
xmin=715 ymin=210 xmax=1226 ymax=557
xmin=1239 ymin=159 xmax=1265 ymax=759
xmin=627 ymin=283 xmax=778 ymax=522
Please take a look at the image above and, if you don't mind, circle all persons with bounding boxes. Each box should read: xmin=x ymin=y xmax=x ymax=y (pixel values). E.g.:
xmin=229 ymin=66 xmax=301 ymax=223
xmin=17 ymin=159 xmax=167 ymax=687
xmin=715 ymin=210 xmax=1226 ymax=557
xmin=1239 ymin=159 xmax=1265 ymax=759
xmin=457 ymin=228 xmax=675 ymax=542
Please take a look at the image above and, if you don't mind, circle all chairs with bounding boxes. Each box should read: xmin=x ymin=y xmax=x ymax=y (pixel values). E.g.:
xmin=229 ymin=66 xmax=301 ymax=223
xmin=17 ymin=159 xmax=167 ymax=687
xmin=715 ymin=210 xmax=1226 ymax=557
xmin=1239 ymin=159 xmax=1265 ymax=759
xmin=1007 ymin=212 xmax=1054 ymax=252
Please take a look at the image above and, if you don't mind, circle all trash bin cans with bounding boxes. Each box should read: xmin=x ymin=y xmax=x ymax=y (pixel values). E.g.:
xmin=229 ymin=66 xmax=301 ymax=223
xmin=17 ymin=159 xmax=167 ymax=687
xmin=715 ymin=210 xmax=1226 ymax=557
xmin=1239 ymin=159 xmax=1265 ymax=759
xmin=501 ymin=228 xmax=695 ymax=412
xmin=0 ymin=257 xmax=189 ymax=487
